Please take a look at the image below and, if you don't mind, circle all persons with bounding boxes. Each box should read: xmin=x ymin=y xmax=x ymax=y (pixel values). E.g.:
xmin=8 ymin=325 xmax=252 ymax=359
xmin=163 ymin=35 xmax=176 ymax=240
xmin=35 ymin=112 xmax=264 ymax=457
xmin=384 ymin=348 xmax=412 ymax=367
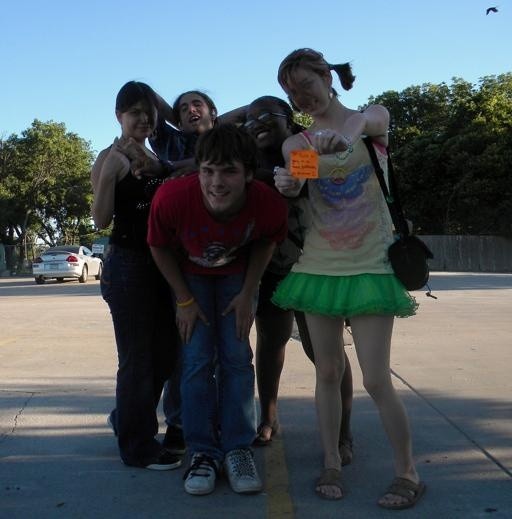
xmin=148 ymin=91 xmax=253 ymax=452
xmin=245 ymin=96 xmax=353 ymax=467
xmin=90 ymin=81 xmax=186 ymax=472
xmin=144 ymin=123 xmax=290 ymax=495
xmin=270 ymin=47 xmax=425 ymax=509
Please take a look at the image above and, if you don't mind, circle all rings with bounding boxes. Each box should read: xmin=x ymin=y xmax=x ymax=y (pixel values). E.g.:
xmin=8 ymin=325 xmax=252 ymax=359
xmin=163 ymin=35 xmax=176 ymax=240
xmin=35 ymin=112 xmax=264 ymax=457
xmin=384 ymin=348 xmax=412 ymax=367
xmin=316 ymin=131 xmax=321 ymax=137
xmin=272 ymin=166 xmax=278 ymax=173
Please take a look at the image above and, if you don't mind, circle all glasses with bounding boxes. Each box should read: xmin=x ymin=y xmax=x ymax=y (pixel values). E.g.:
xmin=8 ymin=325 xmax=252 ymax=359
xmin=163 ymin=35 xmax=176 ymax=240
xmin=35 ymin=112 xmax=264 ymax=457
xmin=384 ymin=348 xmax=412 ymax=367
xmin=243 ymin=111 xmax=286 ymax=129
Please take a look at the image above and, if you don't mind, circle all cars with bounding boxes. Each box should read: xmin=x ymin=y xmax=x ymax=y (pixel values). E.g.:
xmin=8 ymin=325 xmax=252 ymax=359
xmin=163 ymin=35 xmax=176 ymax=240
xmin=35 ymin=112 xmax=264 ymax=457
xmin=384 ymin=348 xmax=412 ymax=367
xmin=33 ymin=245 xmax=104 ymax=284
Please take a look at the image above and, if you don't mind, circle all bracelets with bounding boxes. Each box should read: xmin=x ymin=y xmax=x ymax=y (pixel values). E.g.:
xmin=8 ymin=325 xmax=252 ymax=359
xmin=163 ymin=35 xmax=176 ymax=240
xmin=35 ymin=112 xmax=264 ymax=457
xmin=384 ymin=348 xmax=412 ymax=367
xmin=335 ymin=133 xmax=356 ymax=161
xmin=173 ymin=296 xmax=197 ymax=308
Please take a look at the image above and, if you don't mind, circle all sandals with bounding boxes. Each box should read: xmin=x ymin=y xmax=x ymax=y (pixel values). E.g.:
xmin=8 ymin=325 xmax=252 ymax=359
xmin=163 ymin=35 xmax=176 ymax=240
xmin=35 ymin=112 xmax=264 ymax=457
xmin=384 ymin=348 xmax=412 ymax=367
xmin=338 ymin=435 xmax=355 ymax=465
xmin=257 ymin=419 xmax=279 ymax=447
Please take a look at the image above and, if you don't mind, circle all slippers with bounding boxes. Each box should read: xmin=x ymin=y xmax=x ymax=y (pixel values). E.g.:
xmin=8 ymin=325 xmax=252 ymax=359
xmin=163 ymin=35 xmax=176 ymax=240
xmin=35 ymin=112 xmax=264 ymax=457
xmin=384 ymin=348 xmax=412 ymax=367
xmin=314 ymin=466 xmax=347 ymax=501
xmin=377 ymin=476 xmax=426 ymax=509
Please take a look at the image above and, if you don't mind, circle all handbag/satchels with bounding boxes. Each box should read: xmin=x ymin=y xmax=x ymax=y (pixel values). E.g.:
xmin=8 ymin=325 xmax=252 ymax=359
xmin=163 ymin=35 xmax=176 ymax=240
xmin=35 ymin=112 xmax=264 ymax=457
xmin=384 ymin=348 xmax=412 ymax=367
xmin=385 ymin=217 xmax=434 ymax=292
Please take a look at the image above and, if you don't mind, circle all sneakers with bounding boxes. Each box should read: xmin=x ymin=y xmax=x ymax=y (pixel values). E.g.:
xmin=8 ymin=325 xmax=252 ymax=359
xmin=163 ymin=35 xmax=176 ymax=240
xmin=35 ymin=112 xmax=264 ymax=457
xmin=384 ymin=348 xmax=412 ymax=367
xmin=122 ymin=445 xmax=181 ymax=470
xmin=163 ymin=427 xmax=187 ymax=455
xmin=226 ymin=449 xmax=262 ymax=493
xmin=183 ymin=449 xmax=220 ymax=495
